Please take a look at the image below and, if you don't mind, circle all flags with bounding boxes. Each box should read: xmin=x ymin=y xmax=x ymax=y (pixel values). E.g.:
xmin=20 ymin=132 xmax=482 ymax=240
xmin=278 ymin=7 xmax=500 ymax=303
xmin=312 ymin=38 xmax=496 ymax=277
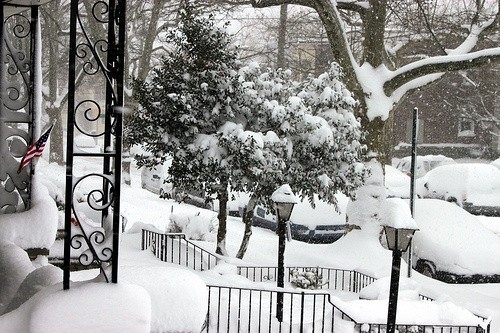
xmin=17 ymin=124 xmax=53 ymax=174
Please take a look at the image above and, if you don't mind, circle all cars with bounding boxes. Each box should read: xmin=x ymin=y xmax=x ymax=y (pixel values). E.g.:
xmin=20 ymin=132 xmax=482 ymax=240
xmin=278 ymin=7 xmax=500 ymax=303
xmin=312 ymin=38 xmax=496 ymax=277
xmin=120 ymin=136 xmax=500 ymax=285
xmin=38 ymin=164 xmax=114 ymax=271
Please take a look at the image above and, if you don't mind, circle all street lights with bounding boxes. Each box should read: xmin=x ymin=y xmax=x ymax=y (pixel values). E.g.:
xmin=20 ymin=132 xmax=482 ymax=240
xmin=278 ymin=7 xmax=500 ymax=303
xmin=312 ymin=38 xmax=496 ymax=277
xmin=380 ymin=211 xmax=420 ymax=333
xmin=270 ymin=185 xmax=297 ymax=321
